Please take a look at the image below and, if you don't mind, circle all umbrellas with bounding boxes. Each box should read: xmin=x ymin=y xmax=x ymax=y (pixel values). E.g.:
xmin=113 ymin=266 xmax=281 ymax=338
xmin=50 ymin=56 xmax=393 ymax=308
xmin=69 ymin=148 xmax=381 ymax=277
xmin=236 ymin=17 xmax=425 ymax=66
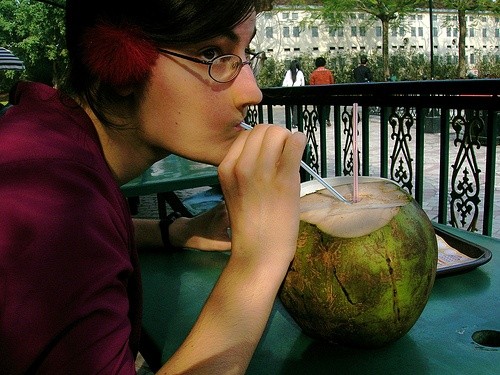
xmin=0 ymin=46 xmax=26 ymax=71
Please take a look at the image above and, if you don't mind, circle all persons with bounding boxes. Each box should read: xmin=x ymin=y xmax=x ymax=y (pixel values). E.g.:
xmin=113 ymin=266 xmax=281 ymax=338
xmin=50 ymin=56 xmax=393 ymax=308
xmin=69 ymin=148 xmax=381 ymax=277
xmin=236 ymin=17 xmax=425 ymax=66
xmin=352 ymin=56 xmax=372 ymax=83
xmin=281 ymin=60 xmax=306 ymax=128
xmin=309 ymin=56 xmax=334 ymax=127
xmin=0 ymin=0 xmax=309 ymax=375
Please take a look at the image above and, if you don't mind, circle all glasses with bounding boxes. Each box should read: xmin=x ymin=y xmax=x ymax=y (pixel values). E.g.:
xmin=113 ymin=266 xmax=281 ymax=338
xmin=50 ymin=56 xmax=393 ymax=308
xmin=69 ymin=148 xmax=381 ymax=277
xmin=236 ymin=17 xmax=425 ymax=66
xmin=156 ymin=47 xmax=268 ymax=83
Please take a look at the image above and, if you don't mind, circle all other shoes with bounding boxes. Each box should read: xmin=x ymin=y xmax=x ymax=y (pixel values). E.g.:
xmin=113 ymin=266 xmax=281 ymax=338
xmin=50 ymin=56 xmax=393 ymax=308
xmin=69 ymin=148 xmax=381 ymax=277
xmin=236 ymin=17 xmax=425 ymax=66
xmin=292 ymin=125 xmax=297 ymax=128
xmin=326 ymin=120 xmax=331 ymax=126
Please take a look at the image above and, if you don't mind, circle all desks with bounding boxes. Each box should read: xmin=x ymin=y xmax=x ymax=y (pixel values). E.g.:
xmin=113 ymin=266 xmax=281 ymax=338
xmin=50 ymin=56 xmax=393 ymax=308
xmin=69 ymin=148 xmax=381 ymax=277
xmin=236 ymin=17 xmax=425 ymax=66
xmin=132 ymin=222 xmax=500 ymax=375
xmin=119 ymin=153 xmax=220 ymax=221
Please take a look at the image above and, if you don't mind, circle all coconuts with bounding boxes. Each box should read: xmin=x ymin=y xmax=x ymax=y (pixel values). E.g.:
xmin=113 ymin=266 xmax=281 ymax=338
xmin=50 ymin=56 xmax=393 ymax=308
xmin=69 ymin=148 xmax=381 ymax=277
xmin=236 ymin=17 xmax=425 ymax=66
xmin=277 ymin=181 xmax=438 ymax=352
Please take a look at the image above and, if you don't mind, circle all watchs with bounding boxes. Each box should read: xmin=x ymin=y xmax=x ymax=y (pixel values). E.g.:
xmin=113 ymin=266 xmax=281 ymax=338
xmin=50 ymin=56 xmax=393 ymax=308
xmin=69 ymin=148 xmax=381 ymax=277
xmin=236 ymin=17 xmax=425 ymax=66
xmin=159 ymin=211 xmax=186 ymax=249
